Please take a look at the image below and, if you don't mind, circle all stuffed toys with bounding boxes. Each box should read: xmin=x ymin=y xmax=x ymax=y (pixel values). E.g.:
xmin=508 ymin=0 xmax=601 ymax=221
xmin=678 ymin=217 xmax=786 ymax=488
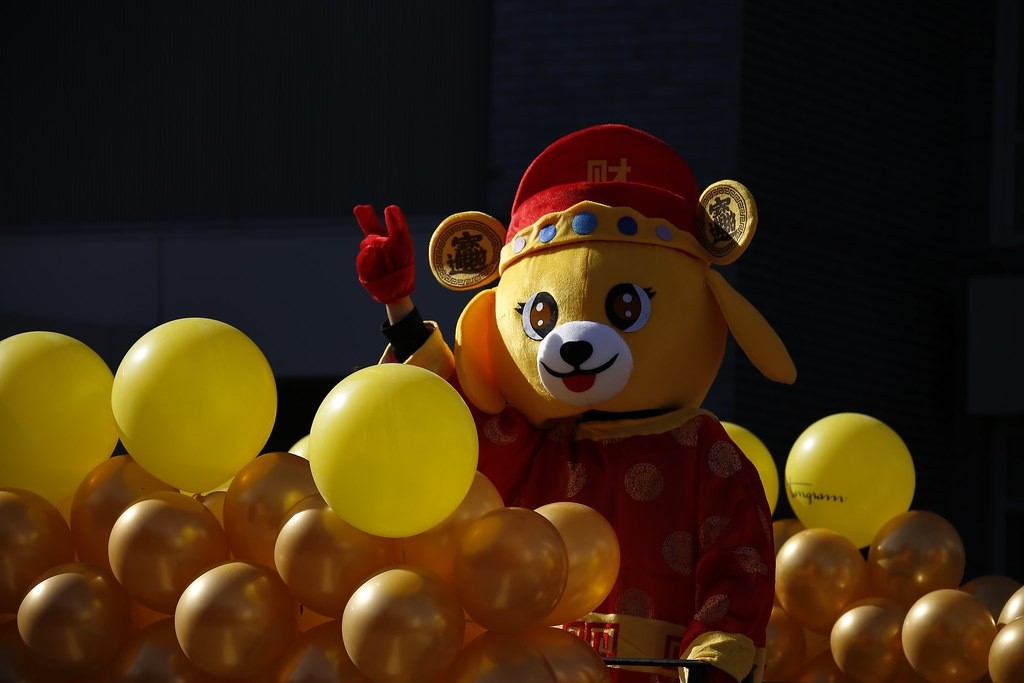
xmin=351 ymin=123 xmax=800 ymax=683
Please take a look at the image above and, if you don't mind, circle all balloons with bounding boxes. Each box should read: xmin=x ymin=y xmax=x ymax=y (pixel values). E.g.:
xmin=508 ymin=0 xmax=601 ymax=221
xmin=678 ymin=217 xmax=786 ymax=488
xmin=1 ymin=316 xmax=1024 ymax=683
xmin=109 ymin=317 xmax=279 ymax=495
xmin=308 ymin=362 xmax=479 ymax=540
xmin=784 ymin=412 xmax=916 ymax=550
